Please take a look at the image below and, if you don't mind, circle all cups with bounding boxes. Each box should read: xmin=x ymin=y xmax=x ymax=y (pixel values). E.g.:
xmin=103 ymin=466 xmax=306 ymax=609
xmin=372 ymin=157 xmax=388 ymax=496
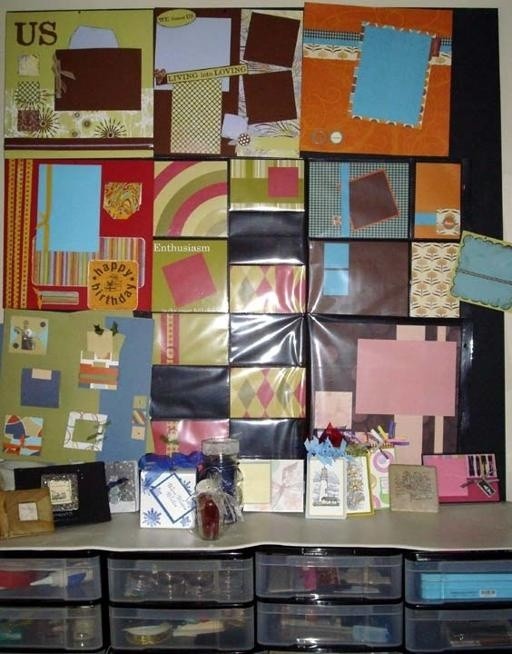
xmin=194 ymin=493 xmax=224 ymax=540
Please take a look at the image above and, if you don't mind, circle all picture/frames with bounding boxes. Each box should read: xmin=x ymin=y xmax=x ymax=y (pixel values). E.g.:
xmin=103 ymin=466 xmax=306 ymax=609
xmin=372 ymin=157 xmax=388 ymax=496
xmin=303 ymin=450 xmax=376 ymax=521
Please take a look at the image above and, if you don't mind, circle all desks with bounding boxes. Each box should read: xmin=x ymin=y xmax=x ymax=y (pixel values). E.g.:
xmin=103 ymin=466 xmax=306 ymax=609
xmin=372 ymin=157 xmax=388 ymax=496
xmin=2 ymin=500 xmax=512 ymax=553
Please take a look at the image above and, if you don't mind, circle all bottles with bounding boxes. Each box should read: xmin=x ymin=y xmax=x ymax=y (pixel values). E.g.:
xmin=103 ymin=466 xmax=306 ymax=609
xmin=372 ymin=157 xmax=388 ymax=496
xmin=198 ymin=438 xmax=241 ymax=525
xmin=30 ymin=562 xmax=94 ymax=588
